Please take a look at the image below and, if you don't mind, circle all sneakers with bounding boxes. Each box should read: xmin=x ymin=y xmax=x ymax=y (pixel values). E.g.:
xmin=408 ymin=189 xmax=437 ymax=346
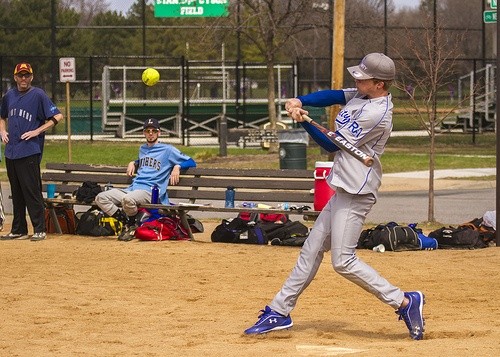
xmin=244 ymin=305 xmax=293 ymax=334
xmin=395 ymin=291 xmax=425 ymax=339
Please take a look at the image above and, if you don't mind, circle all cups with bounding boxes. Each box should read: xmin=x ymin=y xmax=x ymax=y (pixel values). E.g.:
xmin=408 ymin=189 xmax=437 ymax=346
xmin=105 ymin=185 xmax=113 ymax=191
xmin=47 ymin=184 xmax=55 ymax=198
xmin=373 ymin=244 xmax=385 ymax=253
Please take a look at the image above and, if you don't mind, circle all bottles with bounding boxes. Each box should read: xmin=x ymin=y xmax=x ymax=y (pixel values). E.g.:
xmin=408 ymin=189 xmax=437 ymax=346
xmin=151 ymin=184 xmax=159 ymax=204
xmin=277 ymin=202 xmax=299 ymax=210
xmin=225 ymin=184 xmax=235 ymax=207
xmin=241 ymin=201 xmax=258 ymax=208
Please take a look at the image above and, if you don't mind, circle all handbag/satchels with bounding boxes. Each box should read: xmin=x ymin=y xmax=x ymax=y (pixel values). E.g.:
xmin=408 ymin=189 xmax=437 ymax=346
xmin=45 ymin=205 xmax=76 ymax=234
xmin=74 ymin=206 xmax=121 ymax=236
xmin=357 ymin=210 xmax=496 ymax=252
xmin=74 ymin=180 xmax=102 ymax=202
xmin=211 ymin=213 xmax=310 ymax=246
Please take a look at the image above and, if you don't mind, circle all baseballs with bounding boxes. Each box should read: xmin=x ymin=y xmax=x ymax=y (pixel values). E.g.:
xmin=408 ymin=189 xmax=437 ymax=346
xmin=142 ymin=68 xmax=160 ymax=86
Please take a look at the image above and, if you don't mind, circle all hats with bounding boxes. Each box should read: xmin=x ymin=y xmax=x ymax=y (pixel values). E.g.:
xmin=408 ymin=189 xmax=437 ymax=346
xmin=144 ymin=118 xmax=159 ymax=129
xmin=14 ymin=63 xmax=33 ymax=75
xmin=346 ymin=52 xmax=394 ymax=81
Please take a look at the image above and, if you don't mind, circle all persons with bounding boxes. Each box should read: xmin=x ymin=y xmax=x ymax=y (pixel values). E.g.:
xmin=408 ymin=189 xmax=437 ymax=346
xmin=96 ymin=118 xmax=196 ymax=241
xmin=245 ymin=52 xmax=426 ymax=341
xmin=0 ymin=63 xmax=63 ymax=241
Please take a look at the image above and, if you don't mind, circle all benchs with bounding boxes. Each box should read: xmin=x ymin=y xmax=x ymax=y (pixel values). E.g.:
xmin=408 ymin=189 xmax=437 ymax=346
xmin=8 ymin=162 xmax=322 ymax=241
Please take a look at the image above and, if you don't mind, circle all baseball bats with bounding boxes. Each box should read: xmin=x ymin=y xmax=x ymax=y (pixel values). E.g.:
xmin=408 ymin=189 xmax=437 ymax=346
xmin=302 ymin=114 xmax=375 ymax=168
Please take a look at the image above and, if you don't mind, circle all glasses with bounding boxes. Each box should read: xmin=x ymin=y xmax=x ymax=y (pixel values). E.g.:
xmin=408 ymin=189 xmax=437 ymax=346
xmin=146 ymin=129 xmax=159 ymax=133
xmin=18 ymin=73 xmax=31 ymax=78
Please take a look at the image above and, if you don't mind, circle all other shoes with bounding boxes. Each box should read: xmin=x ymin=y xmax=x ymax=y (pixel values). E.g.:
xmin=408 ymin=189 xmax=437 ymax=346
xmin=31 ymin=232 xmax=46 ymax=241
xmin=1 ymin=233 xmax=29 ymax=239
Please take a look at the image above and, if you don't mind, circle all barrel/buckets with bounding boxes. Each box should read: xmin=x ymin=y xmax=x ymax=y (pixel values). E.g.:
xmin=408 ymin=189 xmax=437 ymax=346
xmin=313 ymin=161 xmax=336 ymax=212
